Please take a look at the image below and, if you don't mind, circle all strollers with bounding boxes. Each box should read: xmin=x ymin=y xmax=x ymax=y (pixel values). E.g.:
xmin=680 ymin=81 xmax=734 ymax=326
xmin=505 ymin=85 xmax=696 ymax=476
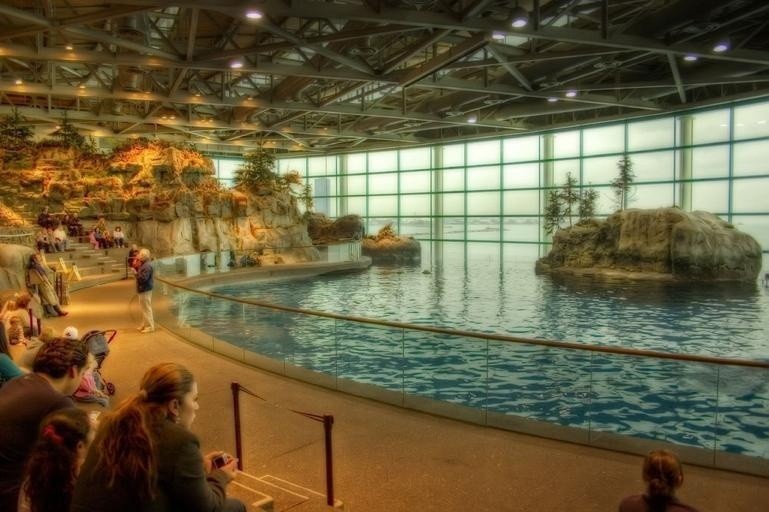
xmin=82 ymin=328 xmax=118 ymax=395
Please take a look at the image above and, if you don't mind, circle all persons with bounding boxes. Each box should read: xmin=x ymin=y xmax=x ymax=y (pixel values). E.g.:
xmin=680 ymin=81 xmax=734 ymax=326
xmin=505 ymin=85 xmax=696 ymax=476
xmin=16 ymin=405 xmax=100 ymax=512
xmin=619 ymin=447 xmax=697 ymax=512
xmin=69 ymin=362 xmax=247 ymax=512
xmin=0 ymin=336 xmax=99 ymax=512
xmin=0 ymin=204 xmax=156 ymax=417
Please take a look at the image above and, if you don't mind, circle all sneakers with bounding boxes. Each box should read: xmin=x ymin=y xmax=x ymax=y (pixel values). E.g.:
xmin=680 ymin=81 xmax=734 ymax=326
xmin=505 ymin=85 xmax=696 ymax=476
xmin=137 ymin=327 xmax=155 ymax=334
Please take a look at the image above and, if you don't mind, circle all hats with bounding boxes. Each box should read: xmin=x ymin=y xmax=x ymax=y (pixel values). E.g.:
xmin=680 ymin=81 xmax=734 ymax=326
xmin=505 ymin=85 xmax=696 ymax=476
xmin=64 ymin=326 xmax=79 ymax=340
